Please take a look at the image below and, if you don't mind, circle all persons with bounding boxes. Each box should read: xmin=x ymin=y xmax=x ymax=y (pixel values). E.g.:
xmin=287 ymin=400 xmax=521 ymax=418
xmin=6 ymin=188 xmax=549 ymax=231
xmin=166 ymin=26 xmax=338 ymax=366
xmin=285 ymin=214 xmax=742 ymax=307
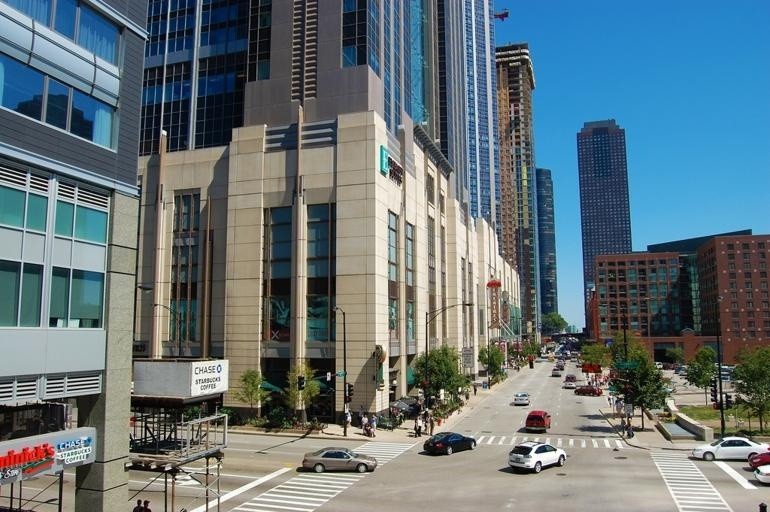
xmin=143 ymin=500 xmax=151 ymax=512
xmin=389 ymin=390 xmax=439 ymax=437
xmin=606 ymin=392 xmax=634 ymax=437
xmin=27 ymin=415 xmax=58 ymax=435
xmin=359 ymin=405 xmax=377 ymax=437
xmin=501 ymin=354 xmax=525 ymax=371
xmin=133 ymin=499 xmax=143 ymax=512
xmin=586 ymin=373 xmax=615 ymax=387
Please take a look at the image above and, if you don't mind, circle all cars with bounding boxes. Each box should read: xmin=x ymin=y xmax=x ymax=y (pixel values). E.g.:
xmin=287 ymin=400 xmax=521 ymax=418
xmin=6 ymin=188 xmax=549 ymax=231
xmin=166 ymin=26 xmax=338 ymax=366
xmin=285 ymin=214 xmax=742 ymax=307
xmin=513 ymin=393 xmax=530 ymax=405
xmin=303 ymin=447 xmax=377 ymax=473
xmin=526 ymin=411 xmax=552 ymax=433
xmin=562 ymin=374 xmax=576 ymax=388
xmin=552 ymin=356 xmax=565 ymax=376
xmin=574 ymin=386 xmax=602 ymax=397
xmin=754 ymin=465 xmax=770 ymax=483
xmin=508 ymin=441 xmax=567 ymax=473
xmin=424 ymin=432 xmax=476 ymax=455
xmin=653 ymin=362 xmax=689 ymax=375
xmin=692 ymin=436 xmax=770 ymax=461
xmin=748 ymin=452 xmax=770 ymax=467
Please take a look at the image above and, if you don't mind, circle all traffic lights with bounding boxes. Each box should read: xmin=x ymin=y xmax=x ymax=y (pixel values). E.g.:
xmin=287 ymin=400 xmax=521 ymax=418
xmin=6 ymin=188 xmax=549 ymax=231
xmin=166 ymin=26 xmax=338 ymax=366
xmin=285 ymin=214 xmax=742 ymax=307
xmin=348 ymin=384 xmax=354 ymax=396
xmin=726 ymin=395 xmax=733 ymax=409
xmin=711 ymin=389 xmax=717 ymax=401
xmin=298 ymin=375 xmax=306 ymax=390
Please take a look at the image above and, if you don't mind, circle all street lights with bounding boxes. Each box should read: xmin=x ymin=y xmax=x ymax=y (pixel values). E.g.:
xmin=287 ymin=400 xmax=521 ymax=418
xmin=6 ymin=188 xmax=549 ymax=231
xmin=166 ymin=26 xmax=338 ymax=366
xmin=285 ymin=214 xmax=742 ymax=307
xmin=487 ymin=317 xmax=523 ymax=388
xmin=333 ymin=307 xmax=347 ymax=435
xmin=714 ymin=295 xmax=724 ymax=437
xmin=426 ymin=304 xmax=474 ymax=407
xmin=153 ymin=303 xmax=182 ymax=357
xmin=599 ymin=304 xmax=628 ymax=402
